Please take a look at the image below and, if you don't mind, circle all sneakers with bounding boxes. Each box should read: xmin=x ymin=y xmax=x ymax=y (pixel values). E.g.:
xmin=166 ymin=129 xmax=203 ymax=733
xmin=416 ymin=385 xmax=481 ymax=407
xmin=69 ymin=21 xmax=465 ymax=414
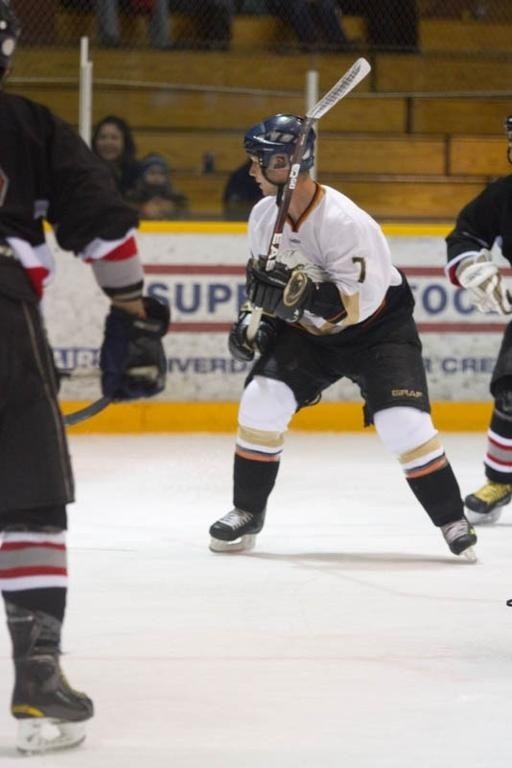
xmin=439 ymin=515 xmax=477 ymax=556
xmin=210 ymin=506 xmax=265 ymax=542
xmin=465 ymin=481 xmax=512 ymax=514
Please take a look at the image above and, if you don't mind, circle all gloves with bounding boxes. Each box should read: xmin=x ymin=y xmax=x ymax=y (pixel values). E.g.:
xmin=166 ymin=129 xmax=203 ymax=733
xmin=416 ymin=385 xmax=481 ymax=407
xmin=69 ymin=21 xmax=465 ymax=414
xmin=454 ymin=248 xmax=511 ymax=313
xmin=244 ymin=253 xmax=319 ymax=323
xmin=228 ymin=298 xmax=282 ymax=361
xmin=96 ymin=297 xmax=171 ymax=401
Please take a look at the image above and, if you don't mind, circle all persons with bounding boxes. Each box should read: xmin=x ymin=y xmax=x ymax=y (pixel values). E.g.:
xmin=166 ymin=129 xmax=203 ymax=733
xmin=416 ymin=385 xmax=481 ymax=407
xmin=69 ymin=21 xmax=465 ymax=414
xmin=1 ymin=5 xmax=171 ymax=732
xmin=172 ymin=1 xmax=233 ymax=51
xmin=438 ymin=113 xmax=512 ymax=513
xmin=267 ymin=1 xmax=353 ymax=53
xmin=199 ymin=109 xmax=479 ymax=558
xmin=123 ymin=140 xmax=191 ymax=224
xmin=221 ymin=158 xmax=265 ymax=225
xmin=94 ymin=0 xmax=175 ymax=50
xmin=91 ymin=109 xmax=140 ymax=200
xmin=343 ymin=0 xmax=423 ymax=53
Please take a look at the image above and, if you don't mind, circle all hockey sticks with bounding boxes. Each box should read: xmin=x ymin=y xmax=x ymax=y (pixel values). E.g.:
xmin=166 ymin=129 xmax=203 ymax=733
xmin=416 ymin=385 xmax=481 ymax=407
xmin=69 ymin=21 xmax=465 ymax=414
xmin=247 ymin=56 xmax=372 ymax=344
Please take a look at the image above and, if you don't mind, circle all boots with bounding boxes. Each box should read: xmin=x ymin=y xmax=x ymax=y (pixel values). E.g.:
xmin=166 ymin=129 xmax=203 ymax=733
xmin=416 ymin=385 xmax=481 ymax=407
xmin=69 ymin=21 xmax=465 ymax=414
xmin=1 ymin=599 xmax=93 ymax=721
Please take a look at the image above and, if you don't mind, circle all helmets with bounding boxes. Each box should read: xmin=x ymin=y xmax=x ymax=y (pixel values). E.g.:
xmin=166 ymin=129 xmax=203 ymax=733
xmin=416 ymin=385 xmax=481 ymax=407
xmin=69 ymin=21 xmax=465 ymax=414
xmin=243 ymin=113 xmax=317 ymax=174
